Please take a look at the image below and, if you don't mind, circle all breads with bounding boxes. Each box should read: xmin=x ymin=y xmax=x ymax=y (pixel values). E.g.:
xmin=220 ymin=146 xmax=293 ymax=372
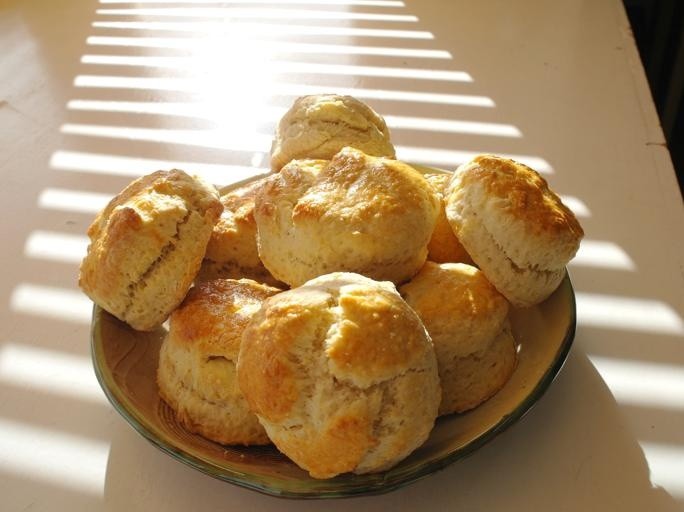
xmin=78 ymin=93 xmax=584 ymax=481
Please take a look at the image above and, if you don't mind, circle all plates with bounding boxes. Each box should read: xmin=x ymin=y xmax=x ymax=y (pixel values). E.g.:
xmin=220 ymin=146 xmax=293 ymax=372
xmin=90 ymin=158 xmax=576 ymax=501
